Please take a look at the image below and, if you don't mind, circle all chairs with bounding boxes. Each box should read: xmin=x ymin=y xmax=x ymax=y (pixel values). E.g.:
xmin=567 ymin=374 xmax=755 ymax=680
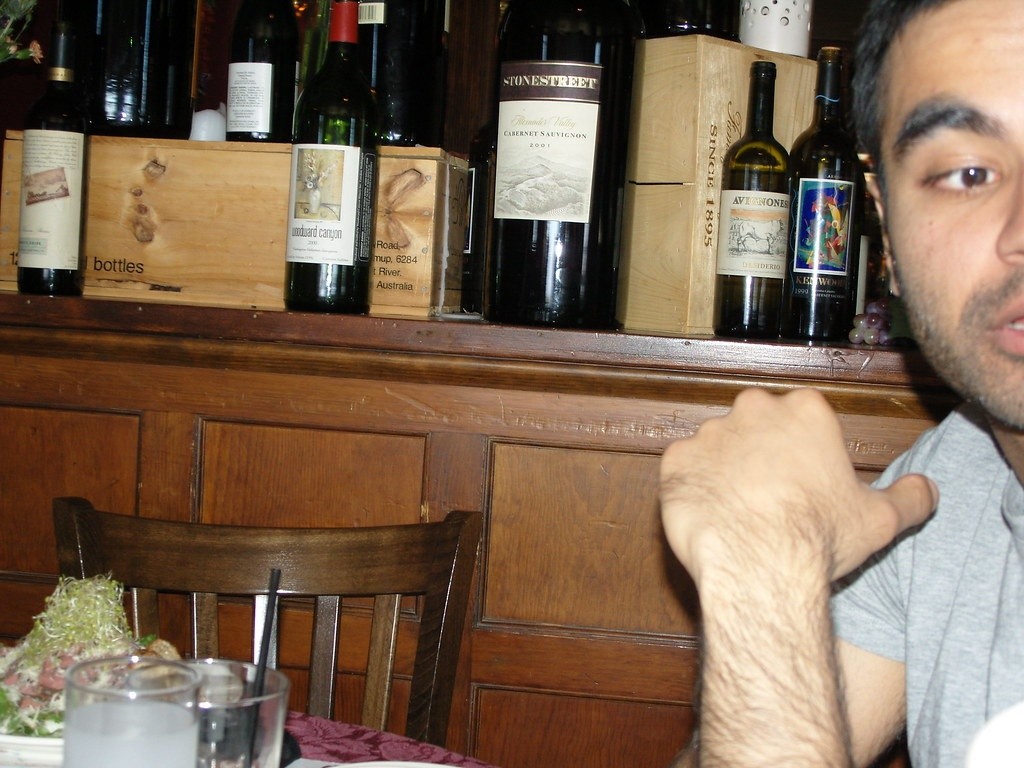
xmin=53 ymin=496 xmax=484 ymax=747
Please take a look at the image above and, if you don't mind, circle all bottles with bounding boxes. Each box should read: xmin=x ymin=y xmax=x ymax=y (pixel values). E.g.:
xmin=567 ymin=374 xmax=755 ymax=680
xmin=782 ymin=45 xmax=871 ymax=347
xmin=16 ymin=19 xmax=89 ymax=296
xmin=283 ymin=0 xmax=376 ymax=314
xmin=356 ymin=0 xmax=452 ymax=149
xmin=226 ymin=0 xmax=302 ymax=143
xmin=459 ymin=0 xmax=743 ymax=332
xmin=715 ymin=60 xmax=794 ymax=339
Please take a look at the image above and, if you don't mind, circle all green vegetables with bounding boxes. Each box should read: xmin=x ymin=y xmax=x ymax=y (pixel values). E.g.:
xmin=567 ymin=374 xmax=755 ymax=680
xmin=0 ymin=684 xmax=67 ymax=739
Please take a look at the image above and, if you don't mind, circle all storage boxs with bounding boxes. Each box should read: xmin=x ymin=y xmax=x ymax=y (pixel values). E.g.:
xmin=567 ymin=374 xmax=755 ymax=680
xmin=368 ymin=145 xmax=469 ymax=323
xmin=616 ymin=35 xmax=818 ymax=341
xmin=0 ymin=126 xmax=292 ymax=311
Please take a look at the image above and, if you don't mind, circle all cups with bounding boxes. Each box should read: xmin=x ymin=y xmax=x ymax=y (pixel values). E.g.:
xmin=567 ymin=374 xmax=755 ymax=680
xmin=63 ymin=655 xmax=205 ymax=768
xmin=171 ymin=657 xmax=291 ymax=768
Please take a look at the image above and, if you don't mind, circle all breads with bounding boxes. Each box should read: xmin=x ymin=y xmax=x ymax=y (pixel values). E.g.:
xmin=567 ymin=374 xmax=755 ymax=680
xmin=127 ymin=637 xmax=184 ymax=683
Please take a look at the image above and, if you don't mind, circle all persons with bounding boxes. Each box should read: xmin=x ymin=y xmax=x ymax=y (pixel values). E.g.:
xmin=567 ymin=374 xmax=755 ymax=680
xmin=659 ymin=0 xmax=1024 ymax=768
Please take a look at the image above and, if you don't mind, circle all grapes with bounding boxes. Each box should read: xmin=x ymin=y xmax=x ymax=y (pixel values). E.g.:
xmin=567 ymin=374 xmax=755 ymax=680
xmin=849 ymin=302 xmax=894 ymax=346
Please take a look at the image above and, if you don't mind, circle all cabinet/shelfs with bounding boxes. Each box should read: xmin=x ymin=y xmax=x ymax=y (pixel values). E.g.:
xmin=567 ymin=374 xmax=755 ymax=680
xmin=0 ymin=1 xmax=964 ymax=768
